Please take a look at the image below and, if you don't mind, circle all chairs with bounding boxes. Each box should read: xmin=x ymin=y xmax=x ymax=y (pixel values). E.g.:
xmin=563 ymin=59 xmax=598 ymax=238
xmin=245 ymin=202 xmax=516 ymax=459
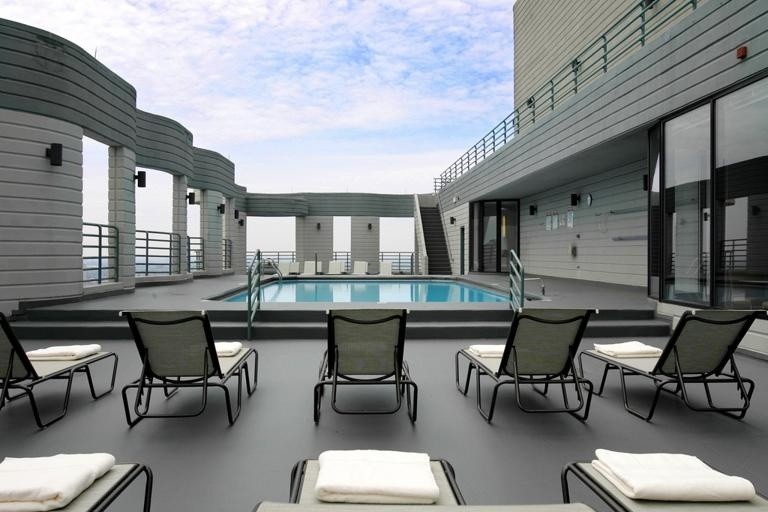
xmin=251 ymin=457 xmax=597 ymax=512
xmin=0 ymin=312 xmax=118 ymax=430
xmin=561 ymin=456 xmax=767 ymax=512
xmin=0 ymin=463 xmax=153 ymax=512
xmin=578 ymin=308 xmax=763 ymax=423
xmin=120 ymin=310 xmax=258 ymax=428
xmin=314 ymin=308 xmax=418 ymax=425
xmin=276 ymin=259 xmax=393 ymax=276
xmin=454 ymin=309 xmax=593 ymax=424
